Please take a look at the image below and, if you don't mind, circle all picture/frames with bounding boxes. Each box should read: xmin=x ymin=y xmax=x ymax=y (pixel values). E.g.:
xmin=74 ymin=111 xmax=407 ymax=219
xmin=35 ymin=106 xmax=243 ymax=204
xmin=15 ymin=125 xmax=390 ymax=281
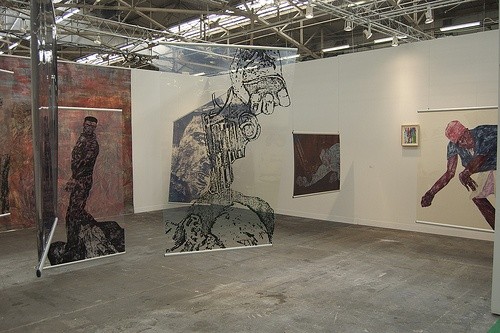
xmin=400 ymin=125 xmax=420 ymax=147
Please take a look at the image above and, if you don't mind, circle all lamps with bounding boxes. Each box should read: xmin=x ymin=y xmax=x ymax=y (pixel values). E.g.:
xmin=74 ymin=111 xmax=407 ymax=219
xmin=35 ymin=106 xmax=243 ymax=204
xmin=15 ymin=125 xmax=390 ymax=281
xmin=21 ymin=21 xmax=28 ymax=33
xmin=94 ymin=36 xmax=102 ymax=45
xmin=391 ymin=37 xmax=398 ymax=47
xmin=344 ymin=22 xmax=352 ymax=31
xmin=363 ymin=28 xmax=372 ymax=39
xmin=305 ymin=6 xmax=313 ymax=19
xmin=424 ymin=13 xmax=434 ymax=24
